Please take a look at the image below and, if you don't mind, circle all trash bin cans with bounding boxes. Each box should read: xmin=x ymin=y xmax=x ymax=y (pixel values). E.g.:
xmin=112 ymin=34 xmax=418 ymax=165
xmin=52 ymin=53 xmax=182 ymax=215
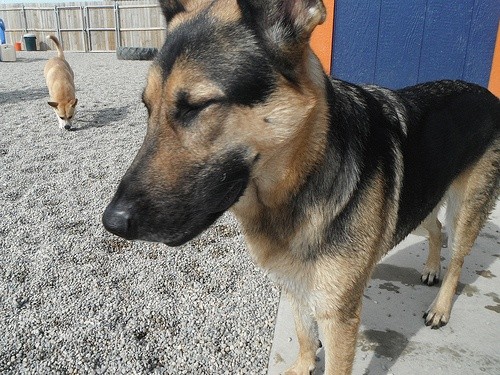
xmin=23 ymin=34 xmax=37 ymax=51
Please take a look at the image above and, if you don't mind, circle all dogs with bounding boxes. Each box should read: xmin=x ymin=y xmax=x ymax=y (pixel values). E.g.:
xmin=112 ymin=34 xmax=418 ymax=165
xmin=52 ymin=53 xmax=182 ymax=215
xmin=43 ymin=35 xmax=79 ymax=132
xmin=98 ymin=0 xmax=500 ymax=375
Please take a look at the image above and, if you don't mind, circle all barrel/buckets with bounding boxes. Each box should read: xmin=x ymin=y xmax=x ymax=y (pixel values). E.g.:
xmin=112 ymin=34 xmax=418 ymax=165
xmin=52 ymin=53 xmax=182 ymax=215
xmin=14 ymin=43 xmax=21 ymax=51
xmin=24 ymin=37 xmax=37 ymax=51
